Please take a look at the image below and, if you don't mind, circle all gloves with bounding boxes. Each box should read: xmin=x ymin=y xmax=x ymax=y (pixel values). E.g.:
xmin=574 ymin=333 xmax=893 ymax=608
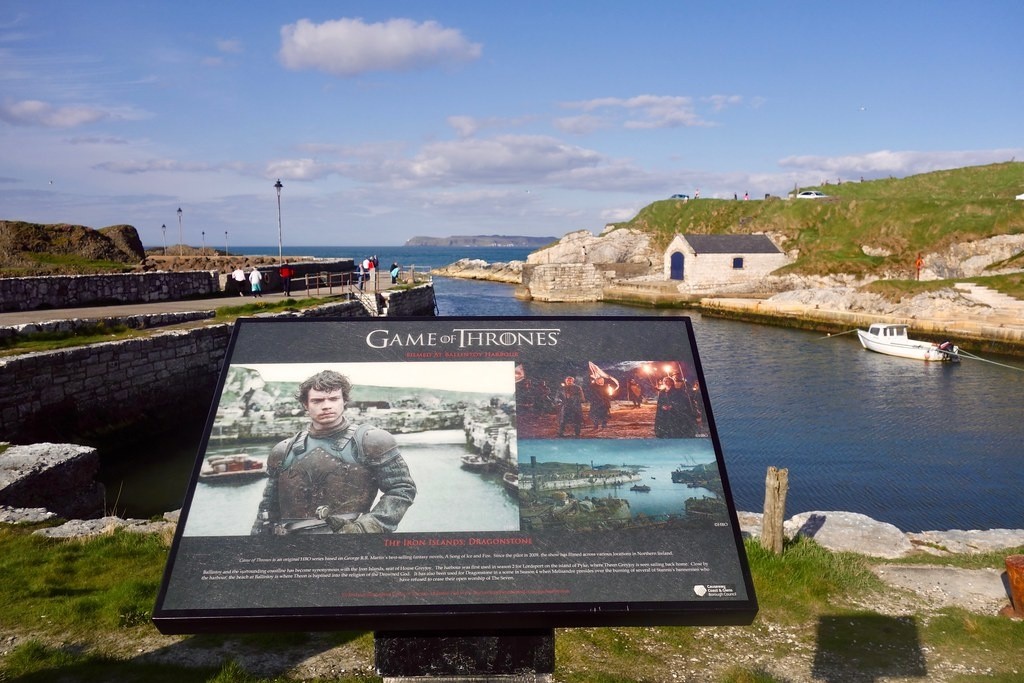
xmin=333 ymin=513 xmax=384 ymax=534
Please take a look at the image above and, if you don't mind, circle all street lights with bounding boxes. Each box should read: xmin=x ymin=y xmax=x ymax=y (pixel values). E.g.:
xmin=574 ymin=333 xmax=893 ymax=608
xmin=177 ymin=207 xmax=183 ymax=259
xmin=162 ymin=224 xmax=167 ymax=257
xmin=274 ymin=178 xmax=284 ymax=265
xmin=202 ymin=231 xmax=206 ymax=255
xmin=224 ymin=231 xmax=228 ymax=257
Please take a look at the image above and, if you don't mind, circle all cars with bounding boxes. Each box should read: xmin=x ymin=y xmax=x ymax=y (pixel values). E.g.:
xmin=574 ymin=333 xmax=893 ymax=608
xmin=671 ymin=194 xmax=689 ymax=201
xmin=797 ymin=190 xmax=829 ymax=199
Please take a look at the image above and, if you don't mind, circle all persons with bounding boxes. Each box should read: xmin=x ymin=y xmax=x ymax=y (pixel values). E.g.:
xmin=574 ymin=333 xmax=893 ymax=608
xmin=356 ymin=263 xmax=365 ymax=290
xmin=250 ymin=369 xmax=416 ymax=536
xmin=249 ymin=266 xmax=262 ymax=298
xmin=389 ymin=260 xmax=400 ymax=284
xmin=519 ymin=355 xmax=706 ymax=440
xmin=233 ymin=265 xmax=246 ymax=296
xmin=279 ymin=259 xmax=294 ymax=297
xmin=363 ymin=254 xmax=379 ymax=275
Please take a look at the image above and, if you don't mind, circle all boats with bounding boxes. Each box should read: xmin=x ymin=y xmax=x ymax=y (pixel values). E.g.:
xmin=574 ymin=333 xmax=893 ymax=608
xmin=198 ymin=450 xmax=265 ymax=481
xmin=630 ymin=484 xmax=652 ymax=494
xmin=460 ymin=452 xmax=497 ymax=471
xmin=501 ymin=471 xmax=519 ymax=492
xmin=856 ymin=322 xmax=959 ymax=361
